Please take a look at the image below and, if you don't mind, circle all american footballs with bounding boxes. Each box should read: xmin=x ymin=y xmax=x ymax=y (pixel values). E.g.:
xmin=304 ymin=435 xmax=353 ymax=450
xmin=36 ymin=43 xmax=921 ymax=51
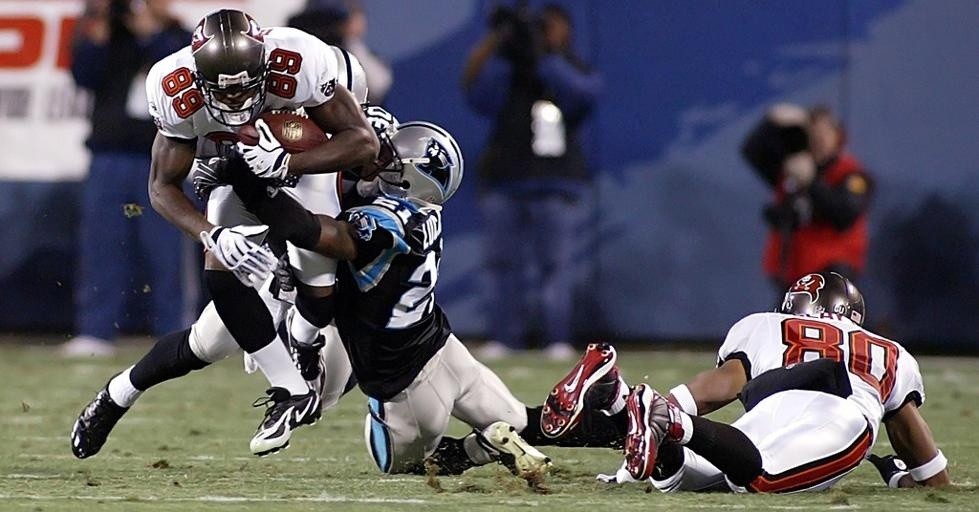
xmin=239 ymin=110 xmax=331 ymax=156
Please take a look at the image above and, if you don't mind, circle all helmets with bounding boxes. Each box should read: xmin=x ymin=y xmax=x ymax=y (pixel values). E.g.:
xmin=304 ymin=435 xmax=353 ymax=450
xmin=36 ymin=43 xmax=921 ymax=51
xmin=777 ymin=271 xmax=866 ymax=330
xmin=380 ymin=119 xmax=466 ymax=207
xmin=191 ymin=8 xmax=268 ymax=127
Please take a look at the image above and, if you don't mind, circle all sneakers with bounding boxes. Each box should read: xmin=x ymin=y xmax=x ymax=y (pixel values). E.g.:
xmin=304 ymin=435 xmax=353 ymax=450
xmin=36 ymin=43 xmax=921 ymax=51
xmin=539 ymin=340 xmax=628 ymax=436
xmin=60 ymin=335 xmax=113 ymax=360
xmin=247 ymin=389 xmax=322 ymax=457
xmin=285 ymin=305 xmax=328 ymax=398
xmin=478 ymin=419 xmax=554 ymax=479
xmin=473 ymin=340 xmax=576 ymax=364
xmin=623 ymin=382 xmax=682 ymax=480
xmin=70 ymin=371 xmax=131 ymax=457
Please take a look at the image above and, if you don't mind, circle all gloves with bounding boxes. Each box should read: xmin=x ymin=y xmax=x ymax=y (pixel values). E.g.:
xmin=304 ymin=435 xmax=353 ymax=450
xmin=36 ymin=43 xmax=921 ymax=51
xmin=868 ymin=450 xmax=911 ymax=488
xmin=214 ymin=149 xmax=248 ymax=188
xmin=233 ymin=115 xmax=292 ymax=179
xmin=200 ymin=223 xmax=275 ymax=287
xmin=363 ymin=103 xmax=400 ymax=141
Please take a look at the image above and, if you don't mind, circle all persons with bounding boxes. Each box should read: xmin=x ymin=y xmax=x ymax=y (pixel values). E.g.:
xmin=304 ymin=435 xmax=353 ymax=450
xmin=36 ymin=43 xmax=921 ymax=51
xmin=741 ymin=103 xmax=879 ymax=313
xmin=244 ymin=46 xmax=399 ymax=411
xmin=458 ymin=0 xmax=602 ymax=362
xmin=54 ymin=0 xmax=203 ymax=360
xmin=540 ymin=271 xmax=950 ymax=493
xmin=192 ymin=121 xmax=623 ymax=479
xmin=71 ymin=9 xmax=382 ymax=460
xmin=303 ymin=0 xmax=393 ymax=106
